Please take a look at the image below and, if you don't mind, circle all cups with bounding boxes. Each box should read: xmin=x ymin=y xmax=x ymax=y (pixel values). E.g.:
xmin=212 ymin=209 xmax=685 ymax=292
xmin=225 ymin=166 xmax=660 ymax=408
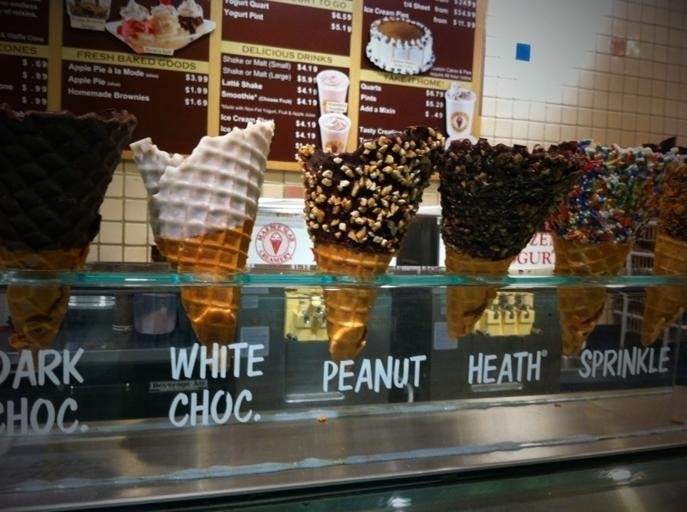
xmin=128 ymin=120 xmax=275 ymax=373
xmin=317 ymin=70 xmax=351 ymax=115
xmin=319 ymin=114 xmax=351 ymax=153
xmin=444 ymin=91 xmax=475 ymax=141
xmin=642 ymin=152 xmax=686 ymax=346
xmin=436 ymin=139 xmax=577 ymax=340
xmin=299 ymin=123 xmax=442 ymax=380
xmin=0 ymin=107 xmax=136 ymax=370
xmin=548 ymin=145 xmax=667 ymax=353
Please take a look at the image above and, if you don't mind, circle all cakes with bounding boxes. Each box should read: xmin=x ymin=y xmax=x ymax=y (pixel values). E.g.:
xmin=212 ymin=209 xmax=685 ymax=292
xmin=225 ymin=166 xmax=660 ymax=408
xmin=121 ymin=15 xmax=146 ymax=44
xmin=148 ymin=4 xmax=178 ymax=38
xmin=365 ymin=15 xmax=437 ymax=76
xmin=118 ymin=0 xmax=150 ymax=22
xmin=176 ymin=0 xmax=205 ymax=32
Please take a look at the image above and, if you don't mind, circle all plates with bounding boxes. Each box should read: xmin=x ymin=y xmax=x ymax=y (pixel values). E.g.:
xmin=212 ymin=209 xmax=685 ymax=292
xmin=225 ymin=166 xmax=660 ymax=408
xmin=106 ymin=21 xmax=217 ymax=52
